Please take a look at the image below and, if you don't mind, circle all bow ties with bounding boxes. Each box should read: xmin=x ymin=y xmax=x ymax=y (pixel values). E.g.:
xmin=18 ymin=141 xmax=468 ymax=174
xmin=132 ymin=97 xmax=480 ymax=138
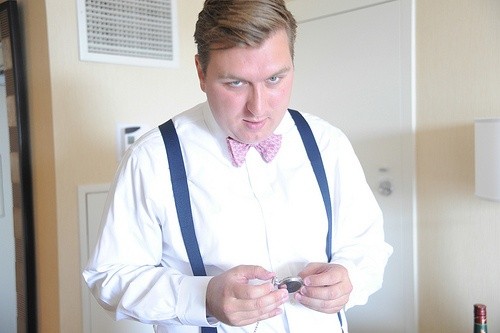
xmin=226 ymin=134 xmax=281 ymax=167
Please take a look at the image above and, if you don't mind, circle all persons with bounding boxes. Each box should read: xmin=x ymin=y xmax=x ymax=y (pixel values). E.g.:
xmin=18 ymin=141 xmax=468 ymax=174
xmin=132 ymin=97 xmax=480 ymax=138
xmin=80 ymin=0 xmax=394 ymax=333
